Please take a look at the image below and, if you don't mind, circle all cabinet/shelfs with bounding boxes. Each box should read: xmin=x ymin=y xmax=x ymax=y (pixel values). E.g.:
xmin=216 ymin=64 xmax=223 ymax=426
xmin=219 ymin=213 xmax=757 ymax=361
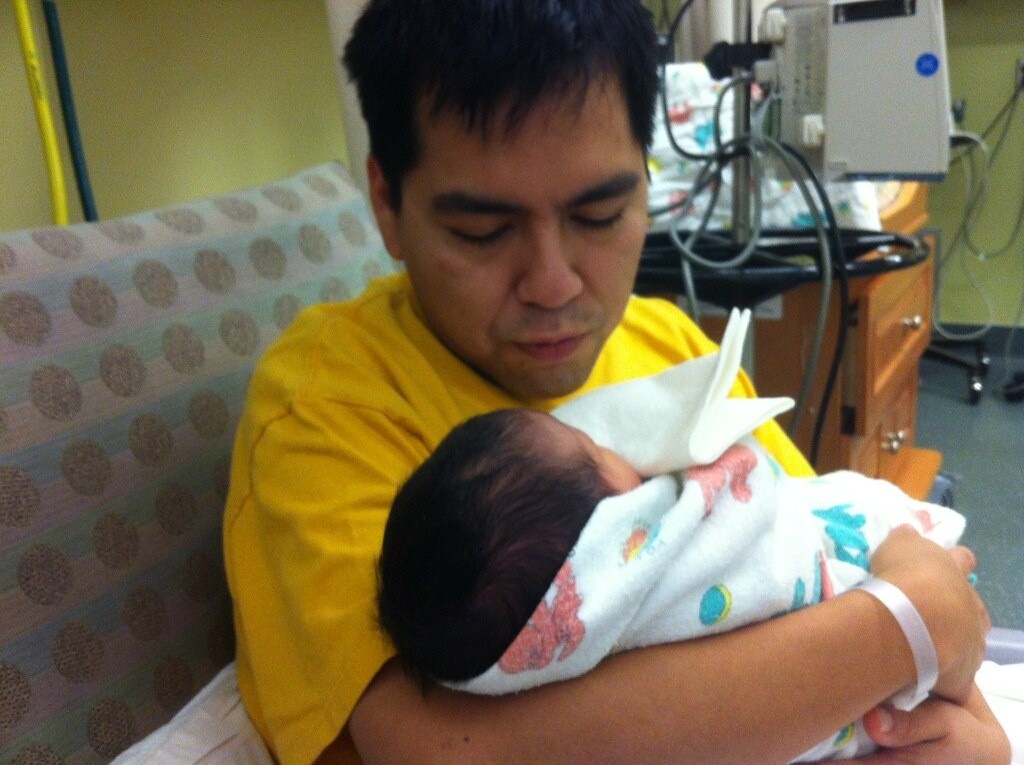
xmin=646 ymin=180 xmax=944 ymax=500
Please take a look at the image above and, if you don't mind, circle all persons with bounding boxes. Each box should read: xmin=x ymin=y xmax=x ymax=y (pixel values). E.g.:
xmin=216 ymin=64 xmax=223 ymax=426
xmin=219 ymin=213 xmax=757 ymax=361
xmin=218 ymin=2 xmax=1012 ymax=764
xmin=370 ymin=407 xmax=971 ymax=760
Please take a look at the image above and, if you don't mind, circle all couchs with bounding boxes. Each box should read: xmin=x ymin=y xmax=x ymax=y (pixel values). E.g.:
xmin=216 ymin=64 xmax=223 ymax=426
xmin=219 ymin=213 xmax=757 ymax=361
xmin=0 ymin=160 xmax=398 ymax=764
